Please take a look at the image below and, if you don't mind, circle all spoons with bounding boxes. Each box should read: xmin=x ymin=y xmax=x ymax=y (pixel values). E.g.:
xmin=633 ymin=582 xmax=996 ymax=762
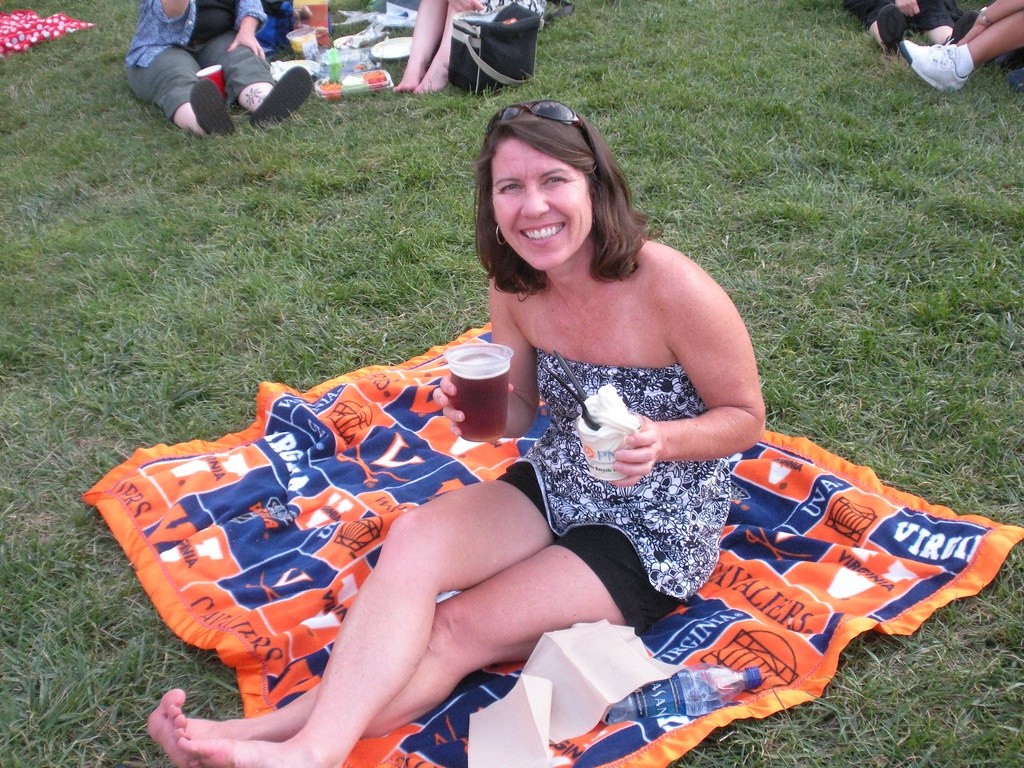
xmin=543 ymin=365 xmax=601 ymax=431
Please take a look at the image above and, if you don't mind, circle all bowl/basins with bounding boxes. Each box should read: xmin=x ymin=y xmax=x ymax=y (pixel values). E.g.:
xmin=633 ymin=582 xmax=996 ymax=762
xmin=314 ymin=68 xmax=395 ymax=102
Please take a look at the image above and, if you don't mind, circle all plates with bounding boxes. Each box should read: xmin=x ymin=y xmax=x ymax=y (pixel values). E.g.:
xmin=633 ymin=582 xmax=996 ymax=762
xmin=332 ymin=35 xmax=389 ymax=49
xmin=371 ymin=36 xmax=411 ymax=60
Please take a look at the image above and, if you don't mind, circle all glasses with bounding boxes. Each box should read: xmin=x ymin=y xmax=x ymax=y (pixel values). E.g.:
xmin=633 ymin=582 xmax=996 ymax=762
xmin=488 ymin=99 xmax=579 ymax=127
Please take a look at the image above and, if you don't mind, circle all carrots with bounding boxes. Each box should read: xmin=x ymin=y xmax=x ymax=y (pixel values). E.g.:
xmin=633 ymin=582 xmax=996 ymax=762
xmin=320 ymin=81 xmax=342 ymax=101
xmin=365 ymin=69 xmax=387 ymax=91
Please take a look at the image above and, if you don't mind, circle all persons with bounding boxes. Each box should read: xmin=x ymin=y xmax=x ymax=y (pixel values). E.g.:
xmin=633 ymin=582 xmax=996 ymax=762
xmin=392 ymin=0 xmax=546 ymax=96
xmin=147 ymin=99 xmax=764 ymax=767
xmin=841 ymin=0 xmax=1024 ymax=94
xmin=125 ymin=0 xmax=315 ymax=135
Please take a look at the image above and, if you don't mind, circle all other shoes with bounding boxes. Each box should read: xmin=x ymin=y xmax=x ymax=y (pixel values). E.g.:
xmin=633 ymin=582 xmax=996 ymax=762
xmin=877 ymin=5 xmax=904 ymax=53
xmin=190 ymin=79 xmax=234 ymax=136
xmin=950 ymin=11 xmax=978 ymax=46
xmin=249 ymin=65 xmax=313 ymax=127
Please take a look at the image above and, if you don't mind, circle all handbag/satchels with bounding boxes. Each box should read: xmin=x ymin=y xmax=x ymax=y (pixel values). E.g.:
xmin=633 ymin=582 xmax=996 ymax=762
xmin=448 ymin=3 xmax=545 ymax=97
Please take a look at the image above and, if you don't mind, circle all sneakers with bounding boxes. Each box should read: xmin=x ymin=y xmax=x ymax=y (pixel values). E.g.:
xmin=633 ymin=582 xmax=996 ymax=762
xmin=899 ymin=40 xmax=968 ymax=93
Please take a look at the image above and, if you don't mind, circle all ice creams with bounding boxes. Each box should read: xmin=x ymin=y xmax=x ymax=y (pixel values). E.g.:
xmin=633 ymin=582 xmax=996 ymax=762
xmin=578 ymin=384 xmax=643 ymax=481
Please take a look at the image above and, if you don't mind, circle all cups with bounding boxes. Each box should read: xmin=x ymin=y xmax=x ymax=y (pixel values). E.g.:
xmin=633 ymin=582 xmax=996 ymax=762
xmin=195 ymin=65 xmax=225 ymax=100
xmin=574 ymin=414 xmax=645 ymax=481
xmin=286 ymin=27 xmax=318 ymax=59
xmin=443 ymin=344 xmax=515 ymax=443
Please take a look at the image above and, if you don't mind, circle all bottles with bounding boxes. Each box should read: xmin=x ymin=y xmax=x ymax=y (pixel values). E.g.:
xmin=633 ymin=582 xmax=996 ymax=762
xmin=612 ymin=667 xmax=761 ymax=725
